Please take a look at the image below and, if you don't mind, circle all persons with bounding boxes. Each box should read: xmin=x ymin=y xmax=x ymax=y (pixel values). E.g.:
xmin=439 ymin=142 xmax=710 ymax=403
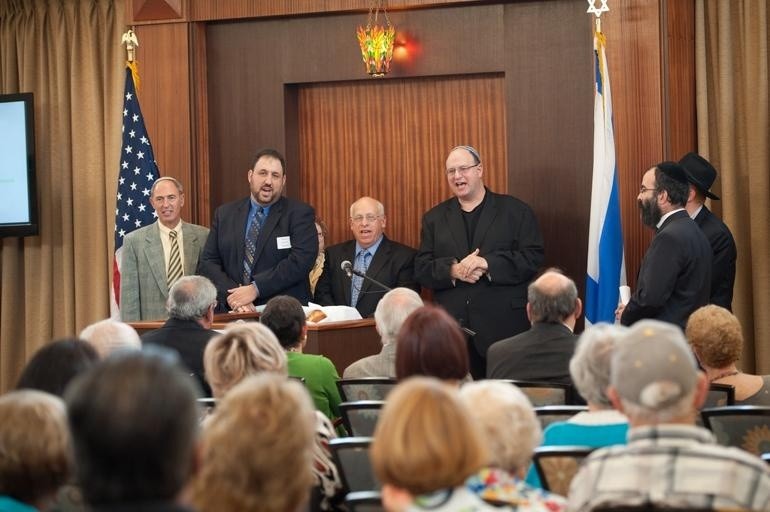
xmin=411 ymin=143 xmax=546 ymax=380
xmin=120 ymin=175 xmax=215 ymax=321
xmin=340 ymin=270 xmax=581 ymax=511
xmin=1 ymin=276 xmax=351 ymax=511
xmin=565 ymin=318 xmax=770 ymax=511
xmin=195 ymin=146 xmax=319 ymax=313
xmin=676 ymin=153 xmax=736 ymax=313
xmin=684 ymin=305 xmax=770 ymax=406
xmin=523 ymin=321 xmax=630 ymax=489
xmin=313 ymin=198 xmax=417 ymax=320
xmin=615 ymin=160 xmax=713 ymax=332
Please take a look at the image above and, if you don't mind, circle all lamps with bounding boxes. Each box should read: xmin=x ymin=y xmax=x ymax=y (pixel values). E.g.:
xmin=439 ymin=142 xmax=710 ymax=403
xmin=356 ymin=0 xmax=395 ymax=76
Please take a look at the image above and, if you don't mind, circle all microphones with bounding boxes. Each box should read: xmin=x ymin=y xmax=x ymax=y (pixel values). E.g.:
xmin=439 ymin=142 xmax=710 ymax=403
xmin=341 ymin=259 xmax=353 ymax=280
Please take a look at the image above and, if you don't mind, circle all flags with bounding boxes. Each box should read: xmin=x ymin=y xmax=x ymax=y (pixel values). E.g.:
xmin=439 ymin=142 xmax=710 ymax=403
xmin=106 ymin=60 xmax=166 ymax=321
xmin=581 ymin=18 xmax=632 ymax=330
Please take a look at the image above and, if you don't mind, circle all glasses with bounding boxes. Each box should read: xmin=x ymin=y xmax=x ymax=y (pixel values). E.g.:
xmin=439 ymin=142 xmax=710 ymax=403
xmin=349 ymin=214 xmax=384 ymax=221
xmin=639 ymin=187 xmax=659 ymax=193
xmin=446 ymin=161 xmax=480 ymax=176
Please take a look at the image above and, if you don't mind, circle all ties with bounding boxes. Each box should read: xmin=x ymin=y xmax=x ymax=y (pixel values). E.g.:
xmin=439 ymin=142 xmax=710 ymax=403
xmin=241 ymin=206 xmax=267 ymax=287
xmin=167 ymin=230 xmax=183 ymax=292
xmin=351 ymin=249 xmax=368 ymax=307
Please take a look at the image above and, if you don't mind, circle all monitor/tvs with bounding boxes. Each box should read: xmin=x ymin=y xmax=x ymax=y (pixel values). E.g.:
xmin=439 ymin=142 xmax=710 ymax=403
xmin=0 ymin=92 xmax=40 ymax=238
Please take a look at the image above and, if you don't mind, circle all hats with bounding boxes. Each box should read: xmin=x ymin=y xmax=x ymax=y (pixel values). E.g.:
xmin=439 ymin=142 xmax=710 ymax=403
xmin=678 ymin=152 xmax=720 ymax=201
xmin=151 ymin=176 xmax=183 ymax=194
xmin=609 ymin=318 xmax=700 ymax=414
xmin=448 ymin=144 xmax=482 ymax=162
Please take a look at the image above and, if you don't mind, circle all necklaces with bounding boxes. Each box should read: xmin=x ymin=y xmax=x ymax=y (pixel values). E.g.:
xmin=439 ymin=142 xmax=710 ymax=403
xmin=710 ymin=368 xmax=742 ymax=382
